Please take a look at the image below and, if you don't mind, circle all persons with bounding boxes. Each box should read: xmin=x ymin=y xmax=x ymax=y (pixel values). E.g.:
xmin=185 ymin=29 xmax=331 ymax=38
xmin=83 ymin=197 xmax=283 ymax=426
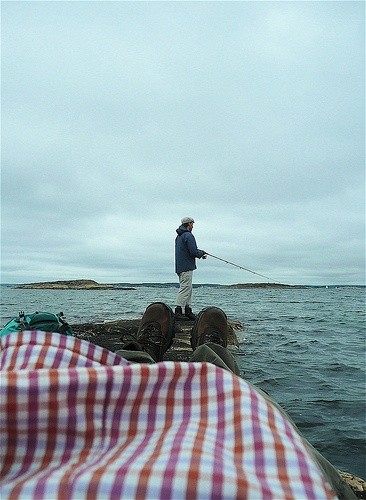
xmin=101 ymin=302 xmax=357 ymax=500
xmin=175 ymin=216 xmax=207 ymax=320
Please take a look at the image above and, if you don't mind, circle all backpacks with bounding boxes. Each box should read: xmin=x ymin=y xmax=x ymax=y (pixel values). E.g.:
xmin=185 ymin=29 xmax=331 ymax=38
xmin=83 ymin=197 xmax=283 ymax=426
xmin=0 ymin=310 xmax=73 ymax=336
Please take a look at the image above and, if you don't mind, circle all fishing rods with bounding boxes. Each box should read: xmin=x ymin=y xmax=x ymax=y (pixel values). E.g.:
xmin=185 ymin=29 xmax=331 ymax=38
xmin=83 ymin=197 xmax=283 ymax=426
xmin=205 ymin=250 xmax=279 ymax=282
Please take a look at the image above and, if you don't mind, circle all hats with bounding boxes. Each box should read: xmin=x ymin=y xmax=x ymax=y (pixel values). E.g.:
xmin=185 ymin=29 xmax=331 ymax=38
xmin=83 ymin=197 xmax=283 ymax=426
xmin=180 ymin=216 xmax=195 ymax=224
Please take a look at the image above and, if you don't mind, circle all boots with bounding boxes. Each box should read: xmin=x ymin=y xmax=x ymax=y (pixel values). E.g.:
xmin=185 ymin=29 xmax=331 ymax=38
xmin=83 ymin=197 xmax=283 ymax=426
xmin=185 ymin=306 xmax=196 ymax=319
xmin=175 ymin=308 xmax=189 ymax=321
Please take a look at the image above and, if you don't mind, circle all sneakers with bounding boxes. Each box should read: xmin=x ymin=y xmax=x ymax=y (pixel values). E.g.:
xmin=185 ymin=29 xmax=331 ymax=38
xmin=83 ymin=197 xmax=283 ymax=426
xmin=189 ymin=305 xmax=228 ymax=351
xmin=121 ymin=300 xmax=176 ymax=363
